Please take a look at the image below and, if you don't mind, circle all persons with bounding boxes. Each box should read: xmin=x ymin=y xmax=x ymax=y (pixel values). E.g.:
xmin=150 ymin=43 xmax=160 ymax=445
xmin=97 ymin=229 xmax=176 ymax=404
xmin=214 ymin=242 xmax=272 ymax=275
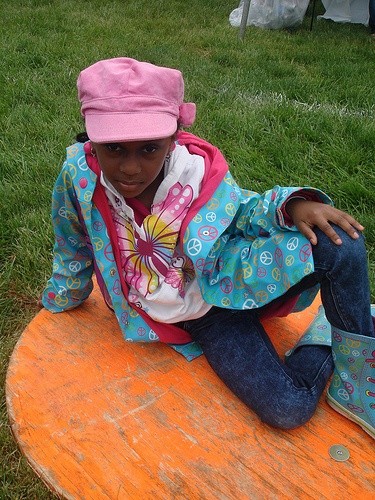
xmin=36 ymin=56 xmax=375 ymax=438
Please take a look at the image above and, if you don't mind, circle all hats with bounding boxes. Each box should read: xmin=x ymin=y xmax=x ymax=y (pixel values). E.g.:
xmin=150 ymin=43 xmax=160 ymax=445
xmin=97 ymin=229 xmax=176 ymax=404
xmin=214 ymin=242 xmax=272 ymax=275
xmin=77 ymin=57 xmax=196 ymax=144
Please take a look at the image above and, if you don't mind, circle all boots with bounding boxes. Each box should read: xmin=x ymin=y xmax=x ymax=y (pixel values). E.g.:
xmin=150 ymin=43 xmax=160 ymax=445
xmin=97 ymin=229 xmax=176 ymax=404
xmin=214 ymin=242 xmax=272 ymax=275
xmin=285 ymin=304 xmax=375 ymax=356
xmin=326 ymin=315 xmax=375 ymax=439
